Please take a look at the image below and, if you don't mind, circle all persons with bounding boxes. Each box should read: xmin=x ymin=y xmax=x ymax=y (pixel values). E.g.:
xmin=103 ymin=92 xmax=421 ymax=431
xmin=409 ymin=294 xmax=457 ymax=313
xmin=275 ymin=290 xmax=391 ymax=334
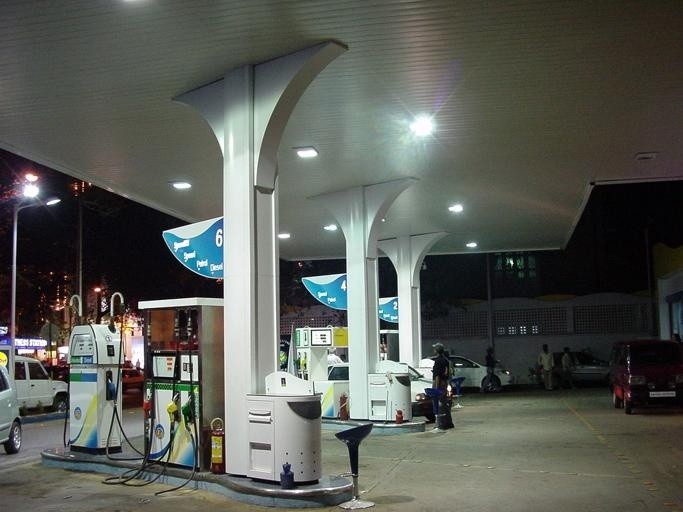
xmin=136 ymin=359 xmax=140 ymax=374
xmin=479 ymin=348 xmax=500 ymax=393
xmin=432 ymin=342 xmax=449 ymax=415
xmin=328 ymin=347 xmax=343 ymax=364
xmin=560 ymin=347 xmax=576 ymax=391
xmin=671 ymin=334 xmax=681 ymax=343
xmin=538 ymin=344 xmax=555 ymax=391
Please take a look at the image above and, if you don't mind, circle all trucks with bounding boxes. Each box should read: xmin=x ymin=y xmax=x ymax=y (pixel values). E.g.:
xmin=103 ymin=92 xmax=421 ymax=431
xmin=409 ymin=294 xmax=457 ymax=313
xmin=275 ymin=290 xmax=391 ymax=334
xmin=56 ymin=345 xmax=70 ymax=365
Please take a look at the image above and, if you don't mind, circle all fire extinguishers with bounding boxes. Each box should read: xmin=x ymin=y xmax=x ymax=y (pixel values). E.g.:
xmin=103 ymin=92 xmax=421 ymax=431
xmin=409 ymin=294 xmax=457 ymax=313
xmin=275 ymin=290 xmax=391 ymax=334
xmin=210 ymin=417 xmax=225 ymax=474
xmin=340 ymin=392 xmax=350 ymax=421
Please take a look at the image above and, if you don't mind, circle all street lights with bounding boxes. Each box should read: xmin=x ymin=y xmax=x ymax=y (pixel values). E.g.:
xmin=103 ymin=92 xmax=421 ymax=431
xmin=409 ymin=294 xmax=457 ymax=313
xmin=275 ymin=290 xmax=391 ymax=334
xmin=9 ymin=186 xmax=62 ymax=384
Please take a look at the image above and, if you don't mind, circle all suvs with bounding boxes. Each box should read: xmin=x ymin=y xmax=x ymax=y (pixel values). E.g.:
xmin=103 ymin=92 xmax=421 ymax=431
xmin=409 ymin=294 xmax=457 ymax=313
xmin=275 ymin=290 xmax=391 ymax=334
xmin=528 ymin=350 xmax=610 ymax=387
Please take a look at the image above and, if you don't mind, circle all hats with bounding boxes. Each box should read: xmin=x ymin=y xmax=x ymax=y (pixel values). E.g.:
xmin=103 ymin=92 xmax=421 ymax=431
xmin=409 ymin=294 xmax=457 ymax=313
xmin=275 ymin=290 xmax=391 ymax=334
xmin=432 ymin=342 xmax=445 ymax=354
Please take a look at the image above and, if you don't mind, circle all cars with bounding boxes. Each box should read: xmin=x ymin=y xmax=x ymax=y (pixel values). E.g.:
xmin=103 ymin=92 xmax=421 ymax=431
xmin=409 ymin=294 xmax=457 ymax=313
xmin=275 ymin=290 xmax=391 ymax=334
xmin=43 ymin=366 xmax=68 ymax=380
xmin=426 ymin=354 xmax=514 ymax=394
xmin=326 ymin=362 xmax=453 ymax=422
xmin=120 ymin=367 xmax=144 ymax=392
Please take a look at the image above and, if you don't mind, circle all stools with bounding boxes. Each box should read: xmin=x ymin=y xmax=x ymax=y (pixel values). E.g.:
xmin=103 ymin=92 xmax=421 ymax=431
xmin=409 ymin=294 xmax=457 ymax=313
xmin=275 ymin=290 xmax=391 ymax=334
xmin=449 ymin=375 xmax=465 ymax=410
xmin=334 ymin=422 xmax=374 ymax=510
xmin=424 ymin=387 xmax=448 ymax=434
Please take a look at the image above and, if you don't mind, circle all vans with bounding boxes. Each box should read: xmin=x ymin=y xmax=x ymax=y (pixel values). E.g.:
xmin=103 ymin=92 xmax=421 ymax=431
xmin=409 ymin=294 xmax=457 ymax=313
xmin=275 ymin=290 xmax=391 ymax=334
xmin=13 ymin=356 xmax=67 ymax=416
xmin=609 ymin=337 xmax=681 ymax=414
xmin=0 ymin=364 xmax=22 ymax=454
